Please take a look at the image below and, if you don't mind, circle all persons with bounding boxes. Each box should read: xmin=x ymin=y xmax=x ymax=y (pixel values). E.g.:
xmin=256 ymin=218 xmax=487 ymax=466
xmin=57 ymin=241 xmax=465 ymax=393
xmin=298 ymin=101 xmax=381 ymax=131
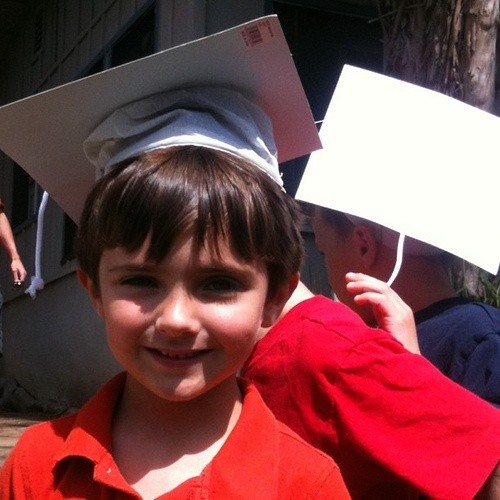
xmin=0 ymin=200 xmax=30 ymax=287
xmin=0 ymin=146 xmax=351 ymax=499
xmin=310 ymin=201 xmax=500 ymax=405
xmin=87 ymin=81 xmax=500 ymax=500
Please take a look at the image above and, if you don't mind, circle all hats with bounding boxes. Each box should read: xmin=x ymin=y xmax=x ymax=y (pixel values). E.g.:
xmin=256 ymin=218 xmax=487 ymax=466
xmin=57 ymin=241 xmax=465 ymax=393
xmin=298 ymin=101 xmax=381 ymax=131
xmin=83 ymin=86 xmax=285 ymax=190
